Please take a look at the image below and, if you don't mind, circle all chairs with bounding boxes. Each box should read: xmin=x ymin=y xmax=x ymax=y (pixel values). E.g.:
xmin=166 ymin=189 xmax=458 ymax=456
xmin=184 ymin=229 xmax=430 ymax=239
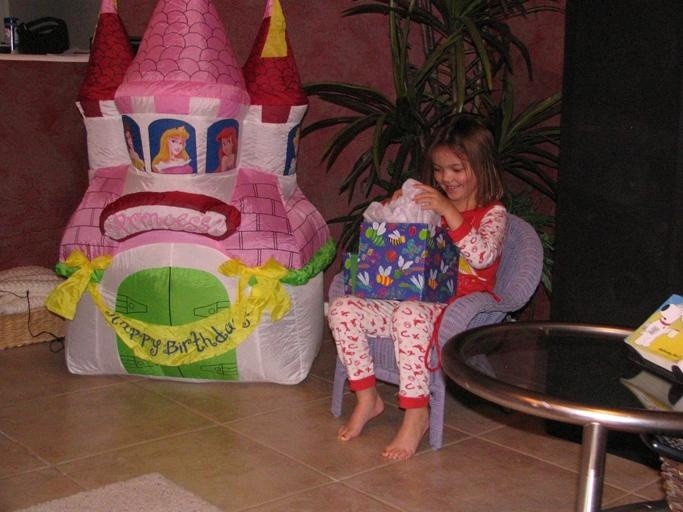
xmin=326 ymin=214 xmax=546 ymax=449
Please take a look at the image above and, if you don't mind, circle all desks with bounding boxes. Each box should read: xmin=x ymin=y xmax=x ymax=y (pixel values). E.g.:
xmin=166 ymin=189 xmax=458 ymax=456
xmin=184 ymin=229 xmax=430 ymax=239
xmin=439 ymin=320 xmax=682 ymax=510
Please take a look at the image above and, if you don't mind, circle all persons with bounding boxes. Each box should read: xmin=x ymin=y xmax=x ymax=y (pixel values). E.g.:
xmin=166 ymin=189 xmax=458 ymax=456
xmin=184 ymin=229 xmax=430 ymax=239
xmin=123 ymin=123 xmax=145 ymax=172
xmin=210 ymin=124 xmax=238 ymax=173
xmin=148 ymin=125 xmax=193 ymax=174
xmin=326 ymin=114 xmax=513 ymax=461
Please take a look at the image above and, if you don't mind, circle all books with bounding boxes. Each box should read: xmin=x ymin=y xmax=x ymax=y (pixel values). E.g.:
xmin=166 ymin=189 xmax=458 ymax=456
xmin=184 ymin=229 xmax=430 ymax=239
xmin=623 ymin=291 xmax=683 ymax=375
xmin=619 ymin=371 xmax=683 ymax=412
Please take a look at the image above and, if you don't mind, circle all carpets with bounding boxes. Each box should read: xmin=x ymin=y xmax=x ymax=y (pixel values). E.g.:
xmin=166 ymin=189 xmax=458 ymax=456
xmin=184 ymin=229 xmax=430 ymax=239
xmin=12 ymin=473 xmax=225 ymax=511
